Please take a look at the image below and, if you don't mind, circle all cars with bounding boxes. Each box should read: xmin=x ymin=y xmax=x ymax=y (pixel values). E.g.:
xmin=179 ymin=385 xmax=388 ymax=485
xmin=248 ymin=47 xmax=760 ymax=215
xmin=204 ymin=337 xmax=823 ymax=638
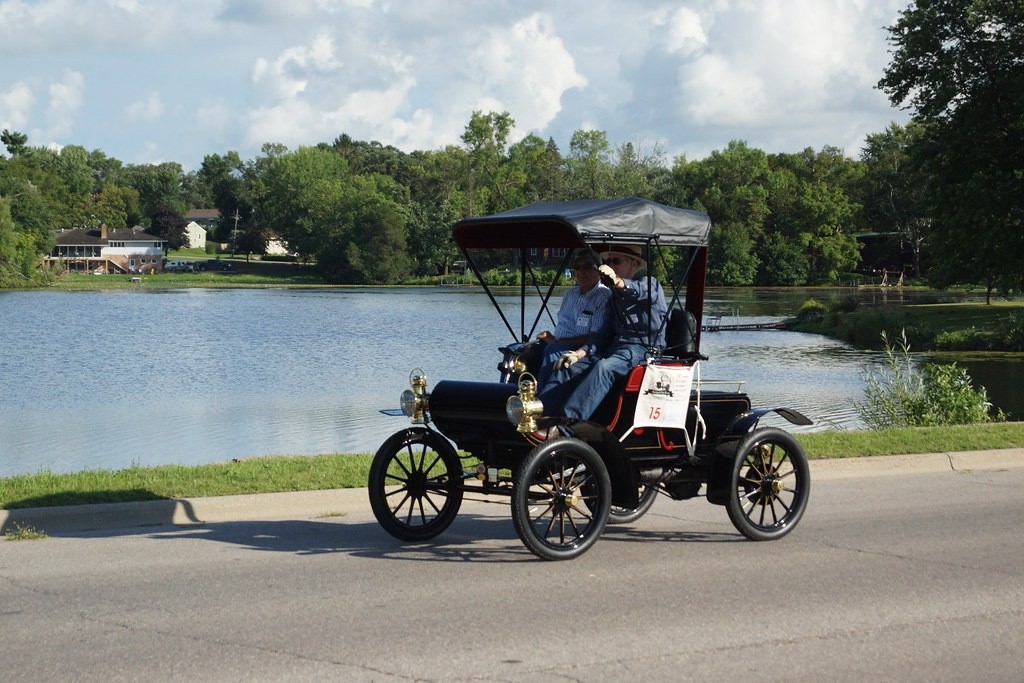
xmin=164 ymin=260 xmax=231 ymax=272
xmin=368 ymin=198 xmax=814 ymax=562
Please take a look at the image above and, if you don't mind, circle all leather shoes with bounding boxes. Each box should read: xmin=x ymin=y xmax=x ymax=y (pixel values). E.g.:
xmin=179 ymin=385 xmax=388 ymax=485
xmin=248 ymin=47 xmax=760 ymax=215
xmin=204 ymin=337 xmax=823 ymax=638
xmin=524 ymin=425 xmax=565 ymax=446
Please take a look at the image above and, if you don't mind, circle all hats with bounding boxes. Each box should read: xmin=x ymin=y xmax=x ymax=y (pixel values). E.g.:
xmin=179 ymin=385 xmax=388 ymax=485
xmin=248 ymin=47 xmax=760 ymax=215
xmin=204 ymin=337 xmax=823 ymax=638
xmin=600 ymin=244 xmax=645 ymax=266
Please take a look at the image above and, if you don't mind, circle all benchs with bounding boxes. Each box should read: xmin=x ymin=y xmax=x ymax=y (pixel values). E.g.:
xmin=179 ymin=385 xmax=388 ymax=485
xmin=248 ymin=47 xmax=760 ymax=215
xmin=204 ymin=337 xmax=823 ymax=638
xmin=586 ymin=307 xmax=697 ymax=429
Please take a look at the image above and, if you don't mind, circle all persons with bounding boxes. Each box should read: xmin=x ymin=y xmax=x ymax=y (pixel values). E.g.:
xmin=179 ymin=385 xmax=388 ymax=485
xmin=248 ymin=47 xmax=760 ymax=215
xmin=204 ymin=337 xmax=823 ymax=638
xmin=517 ymin=244 xmax=669 ymax=447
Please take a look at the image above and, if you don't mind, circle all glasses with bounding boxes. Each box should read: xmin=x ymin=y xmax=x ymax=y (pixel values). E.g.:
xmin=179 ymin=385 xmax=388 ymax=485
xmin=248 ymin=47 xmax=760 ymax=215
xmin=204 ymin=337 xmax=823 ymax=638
xmin=605 ymin=257 xmax=628 ymax=266
xmin=574 ymin=262 xmax=594 ymax=270
